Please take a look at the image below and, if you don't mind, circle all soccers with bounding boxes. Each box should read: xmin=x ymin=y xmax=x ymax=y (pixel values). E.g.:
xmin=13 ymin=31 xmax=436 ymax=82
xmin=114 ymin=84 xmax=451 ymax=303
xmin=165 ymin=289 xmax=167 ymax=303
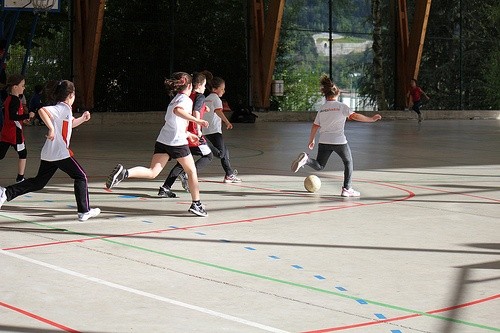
xmin=303 ymin=174 xmax=321 ymax=193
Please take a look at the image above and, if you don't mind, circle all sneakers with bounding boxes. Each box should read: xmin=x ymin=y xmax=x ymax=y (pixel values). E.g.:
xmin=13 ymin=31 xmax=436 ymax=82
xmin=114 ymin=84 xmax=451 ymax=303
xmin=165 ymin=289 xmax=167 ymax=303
xmin=106 ymin=164 xmax=126 ymax=189
xmin=291 ymin=151 xmax=308 ymax=173
xmin=0 ymin=186 xmax=7 ymax=207
xmin=15 ymin=178 xmax=25 ymax=183
xmin=157 ymin=186 xmax=176 ymax=198
xmin=188 ymin=202 xmax=208 ymax=217
xmin=224 ymin=175 xmax=241 ymax=183
xmin=78 ymin=207 xmax=100 ymax=221
xmin=178 ymin=172 xmax=190 ymax=193
xmin=341 ymin=187 xmax=360 ymax=197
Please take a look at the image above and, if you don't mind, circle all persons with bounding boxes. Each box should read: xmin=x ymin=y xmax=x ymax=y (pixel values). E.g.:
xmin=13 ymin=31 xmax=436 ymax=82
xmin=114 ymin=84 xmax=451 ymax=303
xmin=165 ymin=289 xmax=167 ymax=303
xmin=0 ymin=73 xmax=35 ymax=182
xmin=405 ymin=78 xmax=430 ymax=121
xmin=291 ymin=74 xmax=382 ymax=196
xmin=106 ymin=69 xmax=242 ymax=217
xmin=0 ymin=79 xmax=101 ymax=221
